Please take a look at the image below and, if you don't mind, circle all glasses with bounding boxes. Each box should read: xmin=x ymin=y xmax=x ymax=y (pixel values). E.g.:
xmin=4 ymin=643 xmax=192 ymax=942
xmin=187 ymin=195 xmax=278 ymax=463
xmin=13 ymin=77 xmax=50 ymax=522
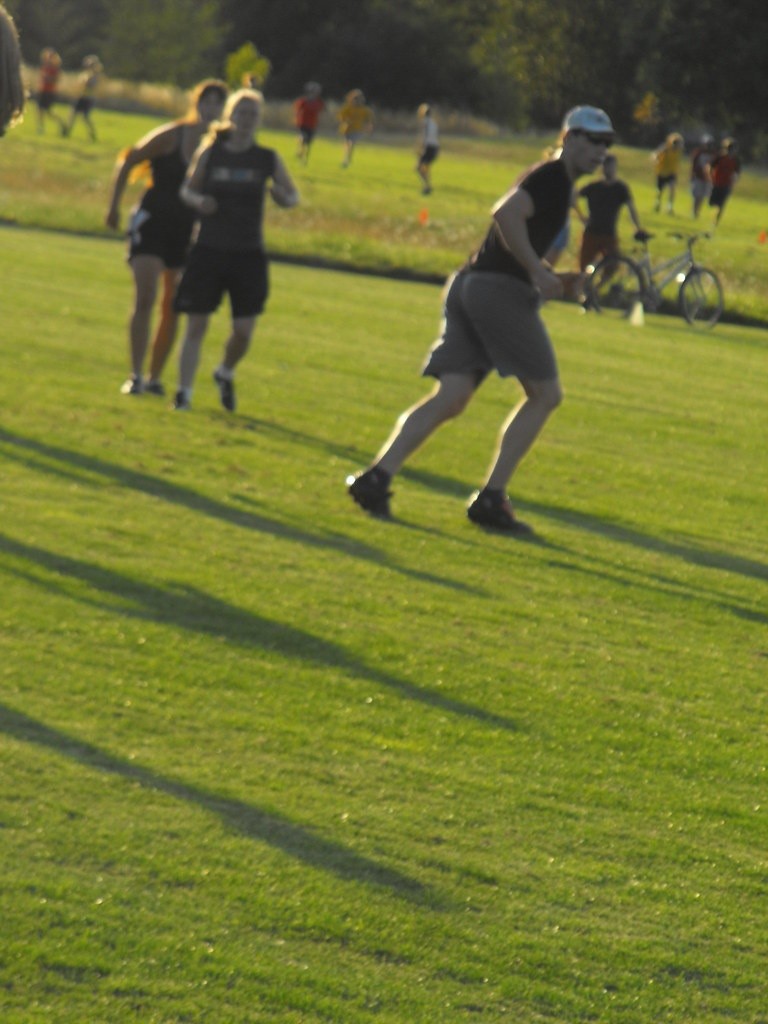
xmin=570 ymin=131 xmax=614 ymax=149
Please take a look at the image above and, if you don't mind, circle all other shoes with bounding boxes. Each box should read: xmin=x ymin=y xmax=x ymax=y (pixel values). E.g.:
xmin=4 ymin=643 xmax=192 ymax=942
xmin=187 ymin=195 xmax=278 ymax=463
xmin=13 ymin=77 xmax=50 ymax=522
xmin=148 ymin=381 xmax=166 ymax=394
xmin=215 ymin=370 xmax=234 ymax=412
xmin=124 ymin=378 xmax=139 ymax=393
xmin=175 ymin=390 xmax=189 ymax=410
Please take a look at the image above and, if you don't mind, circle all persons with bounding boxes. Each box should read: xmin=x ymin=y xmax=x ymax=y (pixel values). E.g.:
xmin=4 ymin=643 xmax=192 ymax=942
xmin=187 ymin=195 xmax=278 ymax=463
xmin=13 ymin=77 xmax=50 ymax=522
xmin=345 ymin=108 xmax=618 ymax=531
xmin=572 ymin=154 xmax=647 ymax=314
xmin=337 ymin=91 xmax=375 ymax=167
xmin=37 ymin=48 xmax=103 ymax=142
xmin=105 ymin=84 xmax=230 ymax=395
xmin=171 ymin=95 xmax=299 ymax=410
xmin=416 ymin=105 xmax=440 ymax=196
xmin=295 ymin=85 xmax=328 ymax=159
xmin=0 ymin=7 xmax=28 ymax=136
xmin=654 ymin=134 xmax=742 ymax=225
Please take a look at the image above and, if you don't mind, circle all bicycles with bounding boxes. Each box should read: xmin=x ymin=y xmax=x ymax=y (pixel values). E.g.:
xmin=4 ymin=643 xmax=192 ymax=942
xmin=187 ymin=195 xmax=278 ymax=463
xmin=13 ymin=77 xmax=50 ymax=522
xmin=582 ymin=229 xmax=724 ymax=331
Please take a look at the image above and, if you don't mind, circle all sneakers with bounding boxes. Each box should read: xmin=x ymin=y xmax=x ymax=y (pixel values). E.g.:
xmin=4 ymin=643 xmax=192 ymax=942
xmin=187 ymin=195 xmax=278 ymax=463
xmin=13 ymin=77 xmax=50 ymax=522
xmin=466 ymin=491 xmax=529 ymax=534
xmin=349 ymin=470 xmax=392 ymax=519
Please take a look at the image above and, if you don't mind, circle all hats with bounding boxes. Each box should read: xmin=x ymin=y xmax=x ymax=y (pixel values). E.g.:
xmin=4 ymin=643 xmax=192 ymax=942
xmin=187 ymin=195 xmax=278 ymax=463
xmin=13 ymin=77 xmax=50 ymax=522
xmin=564 ymin=105 xmax=615 ymax=136
